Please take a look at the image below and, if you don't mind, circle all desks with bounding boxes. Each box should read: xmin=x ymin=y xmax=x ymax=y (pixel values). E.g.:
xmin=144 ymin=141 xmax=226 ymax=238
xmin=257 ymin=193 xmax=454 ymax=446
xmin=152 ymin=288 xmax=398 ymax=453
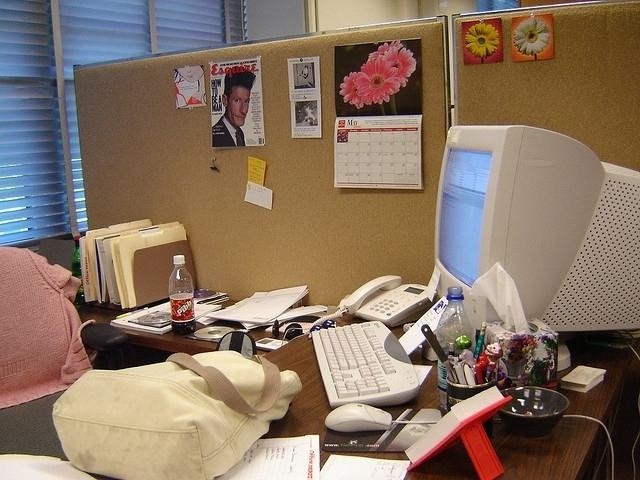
xmin=117 ymin=284 xmax=325 ymax=363
xmin=255 ymin=289 xmax=635 ymax=478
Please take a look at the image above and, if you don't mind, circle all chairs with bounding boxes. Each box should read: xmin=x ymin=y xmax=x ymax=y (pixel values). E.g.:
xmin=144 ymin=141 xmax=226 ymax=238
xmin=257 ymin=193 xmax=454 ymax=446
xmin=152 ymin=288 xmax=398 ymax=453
xmin=0 ymin=245 xmax=98 ymax=462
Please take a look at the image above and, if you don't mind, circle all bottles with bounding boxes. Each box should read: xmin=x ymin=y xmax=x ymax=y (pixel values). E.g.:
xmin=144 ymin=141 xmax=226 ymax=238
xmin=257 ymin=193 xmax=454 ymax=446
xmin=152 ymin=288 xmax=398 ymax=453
xmin=72 ymin=235 xmax=88 ymax=304
xmin=436 ymin=285 xmax=476 ymax=410
xmin=168 ymin=255 xmax=196 ymax=333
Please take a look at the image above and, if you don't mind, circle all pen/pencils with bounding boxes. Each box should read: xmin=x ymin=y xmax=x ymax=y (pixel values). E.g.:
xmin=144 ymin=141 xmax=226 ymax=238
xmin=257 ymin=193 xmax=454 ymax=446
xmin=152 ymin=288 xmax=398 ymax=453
xmin=393 ymin=421 xmax=437 ymax=424
xmin=443 ymin=322 xmax=502 ymax=387
xmin=116 ymin=307 xmax=149 ymax=319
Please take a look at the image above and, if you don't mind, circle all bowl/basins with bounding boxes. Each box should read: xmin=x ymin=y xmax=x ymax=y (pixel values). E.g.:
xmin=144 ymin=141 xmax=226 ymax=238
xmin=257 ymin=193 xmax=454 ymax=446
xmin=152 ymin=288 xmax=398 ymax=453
xmin=497 ymin=387 xmax=570 ymax=435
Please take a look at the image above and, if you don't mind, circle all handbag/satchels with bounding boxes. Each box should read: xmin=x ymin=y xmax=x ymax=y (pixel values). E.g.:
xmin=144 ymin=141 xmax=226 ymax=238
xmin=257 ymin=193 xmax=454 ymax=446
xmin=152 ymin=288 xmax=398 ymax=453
xmin=48 ymin=346 xmax=303 ymax=477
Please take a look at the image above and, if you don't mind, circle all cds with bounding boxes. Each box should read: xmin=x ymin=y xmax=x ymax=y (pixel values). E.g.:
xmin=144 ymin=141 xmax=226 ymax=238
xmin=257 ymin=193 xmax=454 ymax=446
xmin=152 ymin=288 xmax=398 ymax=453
xmin=194 ymin=326 xmax=236 ymax=339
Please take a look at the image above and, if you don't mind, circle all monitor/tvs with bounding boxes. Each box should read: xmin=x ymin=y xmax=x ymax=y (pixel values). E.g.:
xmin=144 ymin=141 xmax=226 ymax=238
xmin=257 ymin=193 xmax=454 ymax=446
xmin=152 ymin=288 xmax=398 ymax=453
xmin=432 ymin=124 xmax=640 ymax=332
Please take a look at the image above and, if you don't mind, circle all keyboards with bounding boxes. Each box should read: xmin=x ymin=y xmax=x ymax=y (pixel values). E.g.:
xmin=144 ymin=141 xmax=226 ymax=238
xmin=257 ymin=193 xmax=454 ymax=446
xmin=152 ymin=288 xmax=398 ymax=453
xmin=310 ymin=320 xmax=420 ymax=407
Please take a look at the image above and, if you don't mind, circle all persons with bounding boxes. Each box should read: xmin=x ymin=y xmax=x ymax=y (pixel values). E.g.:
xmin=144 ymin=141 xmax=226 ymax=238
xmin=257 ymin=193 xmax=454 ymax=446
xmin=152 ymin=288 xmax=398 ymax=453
xmin=212 ymin=71 xmax=256 ymax=147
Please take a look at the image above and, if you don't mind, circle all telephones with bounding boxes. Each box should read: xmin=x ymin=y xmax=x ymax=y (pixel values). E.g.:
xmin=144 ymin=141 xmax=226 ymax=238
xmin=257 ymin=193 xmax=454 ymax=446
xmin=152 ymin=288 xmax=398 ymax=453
xmin=345 ymin=274 xmax=438 ymax=327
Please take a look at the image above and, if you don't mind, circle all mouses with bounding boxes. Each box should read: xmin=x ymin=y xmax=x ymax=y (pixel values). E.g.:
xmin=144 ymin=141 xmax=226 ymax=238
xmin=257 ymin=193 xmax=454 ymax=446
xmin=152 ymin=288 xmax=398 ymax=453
xmin=324 ymin=403 xmax=393 ymax=432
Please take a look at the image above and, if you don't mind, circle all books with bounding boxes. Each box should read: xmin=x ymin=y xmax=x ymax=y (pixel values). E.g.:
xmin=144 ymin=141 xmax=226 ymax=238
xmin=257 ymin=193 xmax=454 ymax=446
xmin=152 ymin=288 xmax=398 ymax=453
xmin=79 ymin=219 xmax=197 ymax=309
xmin=111 ymin=300 xmax=222 ymax=334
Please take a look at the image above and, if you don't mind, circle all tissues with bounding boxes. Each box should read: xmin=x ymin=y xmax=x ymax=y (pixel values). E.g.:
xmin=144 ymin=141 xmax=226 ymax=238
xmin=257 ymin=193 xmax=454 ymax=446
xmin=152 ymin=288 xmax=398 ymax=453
xmin=469 ymin=261 xmax=558 ymax=391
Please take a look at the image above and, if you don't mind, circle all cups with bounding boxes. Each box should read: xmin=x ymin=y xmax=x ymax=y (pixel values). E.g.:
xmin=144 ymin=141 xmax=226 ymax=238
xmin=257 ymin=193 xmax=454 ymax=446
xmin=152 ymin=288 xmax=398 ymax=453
xmin=446 ymin=376 xmax=496 ymax=414
